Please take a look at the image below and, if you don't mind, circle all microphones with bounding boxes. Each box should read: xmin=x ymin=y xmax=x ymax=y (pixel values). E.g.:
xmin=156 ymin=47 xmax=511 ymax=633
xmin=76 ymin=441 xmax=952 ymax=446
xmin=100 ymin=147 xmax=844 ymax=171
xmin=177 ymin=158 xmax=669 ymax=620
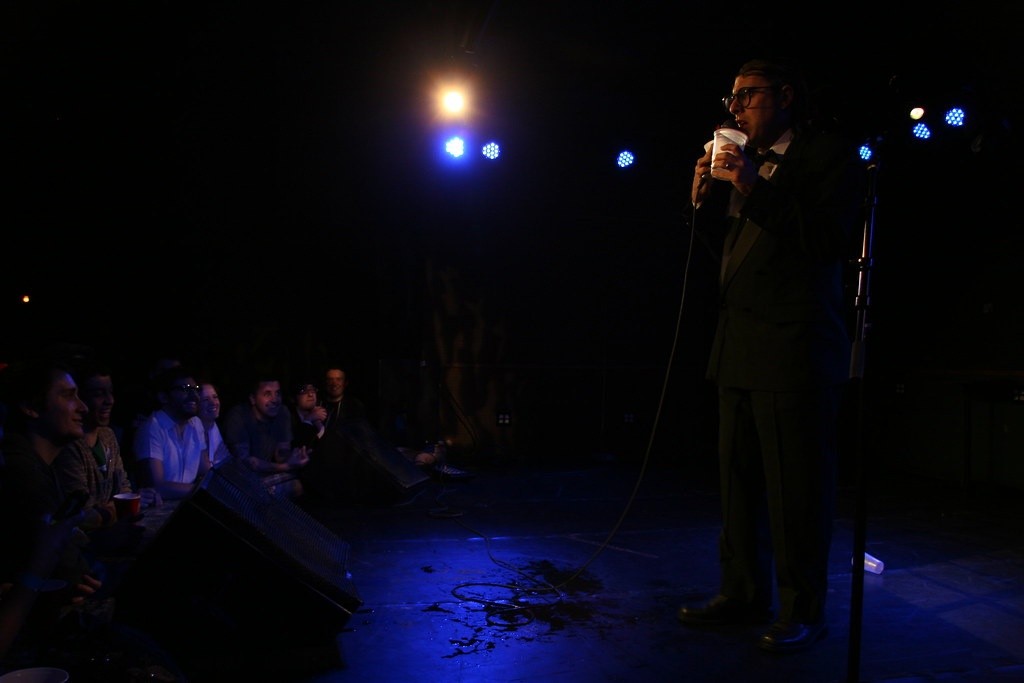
xmin=697 ymin=121 xmax=733 ymax=190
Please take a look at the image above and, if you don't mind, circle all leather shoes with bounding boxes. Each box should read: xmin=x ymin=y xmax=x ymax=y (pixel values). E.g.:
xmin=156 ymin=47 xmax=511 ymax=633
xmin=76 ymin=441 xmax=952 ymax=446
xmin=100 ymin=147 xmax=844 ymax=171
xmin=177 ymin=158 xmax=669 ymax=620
xmin=677 ymin=593 xmax=774 ymax=627
xmin=758 ymin=619 xmax=829 ymax=655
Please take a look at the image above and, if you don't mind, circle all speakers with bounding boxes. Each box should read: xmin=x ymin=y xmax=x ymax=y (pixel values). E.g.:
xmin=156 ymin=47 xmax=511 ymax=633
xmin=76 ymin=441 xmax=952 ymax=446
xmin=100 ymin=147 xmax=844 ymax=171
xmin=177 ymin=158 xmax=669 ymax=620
xmin=157 ymin=456 xmax=364 ymax=646
xmin=314 ymin=418 xmax=431 ymax=509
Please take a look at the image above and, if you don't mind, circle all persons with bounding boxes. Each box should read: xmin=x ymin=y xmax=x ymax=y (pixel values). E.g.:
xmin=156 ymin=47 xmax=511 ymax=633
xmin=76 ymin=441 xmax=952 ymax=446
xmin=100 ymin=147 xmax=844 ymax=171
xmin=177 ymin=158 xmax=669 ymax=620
xmin=51 ymin=369 xmax=135 ymax=533
xmin=284 ymin=375 xmax=354 ymax=480
xmin=678 ymin=62 xmax=838 ymax=658
xmin=187 ymin=381 xmax=234 ymax=466
xmin=132 ymin=374 xmax=212 ymax=500
xmin=0 ymin=363 xmax=116 ymax=620
xmin=227 ymin=374 xmax=309 ymax=496
xmin=0 ymin=455 xmax=192 ymax=683
xmin=319 ymin=362 xmax=365 ymax=440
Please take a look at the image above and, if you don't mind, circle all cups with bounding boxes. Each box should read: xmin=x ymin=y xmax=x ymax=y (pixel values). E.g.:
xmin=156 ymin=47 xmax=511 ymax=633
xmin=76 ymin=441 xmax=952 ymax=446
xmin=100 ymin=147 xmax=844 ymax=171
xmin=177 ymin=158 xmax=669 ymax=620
xmin=850 ymin=550 xmax=885 ymax=575
xmin=0 ymin=668 xmax=70 ymax=683
xmin=113 ymin=494 xmax=141 ymax=521
xmin=712 ymin=129 xmax=748 ymax=181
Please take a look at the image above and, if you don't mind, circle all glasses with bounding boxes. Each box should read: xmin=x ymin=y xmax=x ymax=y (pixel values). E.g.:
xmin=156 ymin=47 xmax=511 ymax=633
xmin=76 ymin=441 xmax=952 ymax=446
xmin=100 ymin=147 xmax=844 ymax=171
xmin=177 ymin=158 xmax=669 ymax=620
xmin=178 ymin=382 xmax=201 ymax=393
xmin=721 ymin=85 xmax=774 ymax=109
xmin=197 ymin=393 xmax=220 ymax=404
xmin=296 ymin=387 xmax=318 ymax=395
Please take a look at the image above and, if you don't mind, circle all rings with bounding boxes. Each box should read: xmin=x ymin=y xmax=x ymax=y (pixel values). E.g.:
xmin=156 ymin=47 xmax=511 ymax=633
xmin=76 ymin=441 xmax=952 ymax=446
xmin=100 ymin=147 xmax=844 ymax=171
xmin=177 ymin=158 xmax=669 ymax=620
xmin=725 ymin=162 xmax=731 ymax=169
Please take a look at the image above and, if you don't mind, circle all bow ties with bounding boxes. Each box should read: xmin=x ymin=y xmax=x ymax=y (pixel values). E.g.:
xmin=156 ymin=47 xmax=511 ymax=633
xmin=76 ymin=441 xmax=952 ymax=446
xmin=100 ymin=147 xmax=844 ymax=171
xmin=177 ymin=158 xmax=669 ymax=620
xmin=751 ymin=149 xmax=779 ymax=169
